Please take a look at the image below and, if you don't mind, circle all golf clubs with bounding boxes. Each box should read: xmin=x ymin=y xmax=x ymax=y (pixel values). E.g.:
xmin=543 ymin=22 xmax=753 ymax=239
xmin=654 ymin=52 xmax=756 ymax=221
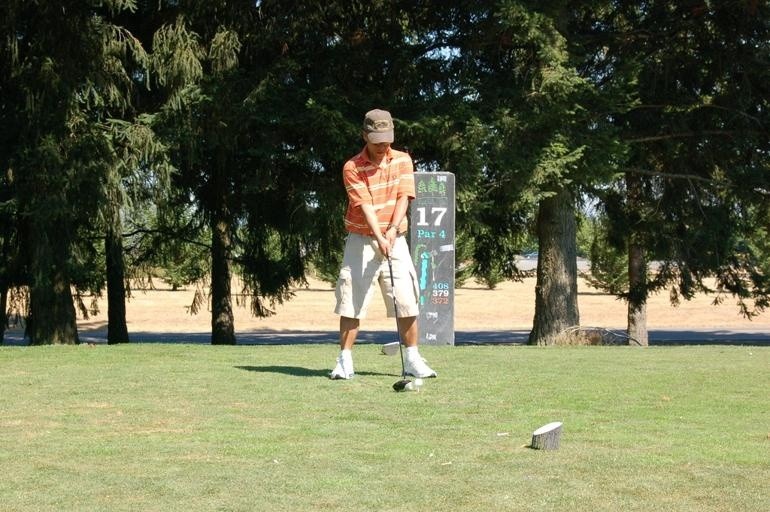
xmin=384 ymin=231 xmax=413 ymax=392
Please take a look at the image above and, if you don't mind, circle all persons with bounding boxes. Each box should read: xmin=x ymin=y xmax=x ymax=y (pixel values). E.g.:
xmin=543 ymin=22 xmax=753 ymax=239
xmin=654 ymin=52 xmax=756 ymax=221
xmin=328 ymin=109 xmax=438 ymax=380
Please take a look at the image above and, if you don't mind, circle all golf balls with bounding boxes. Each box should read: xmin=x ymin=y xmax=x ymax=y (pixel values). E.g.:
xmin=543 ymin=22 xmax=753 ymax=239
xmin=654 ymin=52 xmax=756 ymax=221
xmin=415 ymin=379 xmax=423 ymax=386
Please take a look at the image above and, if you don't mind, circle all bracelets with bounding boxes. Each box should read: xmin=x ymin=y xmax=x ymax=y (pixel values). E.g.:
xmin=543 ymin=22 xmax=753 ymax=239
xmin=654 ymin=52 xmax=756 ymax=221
xmin=387 ymin=224 xmax=400 ymax=234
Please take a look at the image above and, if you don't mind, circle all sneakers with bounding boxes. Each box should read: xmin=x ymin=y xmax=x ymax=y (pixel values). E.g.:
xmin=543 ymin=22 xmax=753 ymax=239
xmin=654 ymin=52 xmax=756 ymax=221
xmin=402 ymin=345 xmax=438 ymax=377
xmin=330 ymin=348 xmax=355 ymax=380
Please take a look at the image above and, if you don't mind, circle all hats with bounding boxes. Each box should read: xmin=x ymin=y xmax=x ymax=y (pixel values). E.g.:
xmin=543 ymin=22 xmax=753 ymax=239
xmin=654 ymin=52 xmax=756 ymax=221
xmin=362 ymin=108 xmax=395 ymax=144
